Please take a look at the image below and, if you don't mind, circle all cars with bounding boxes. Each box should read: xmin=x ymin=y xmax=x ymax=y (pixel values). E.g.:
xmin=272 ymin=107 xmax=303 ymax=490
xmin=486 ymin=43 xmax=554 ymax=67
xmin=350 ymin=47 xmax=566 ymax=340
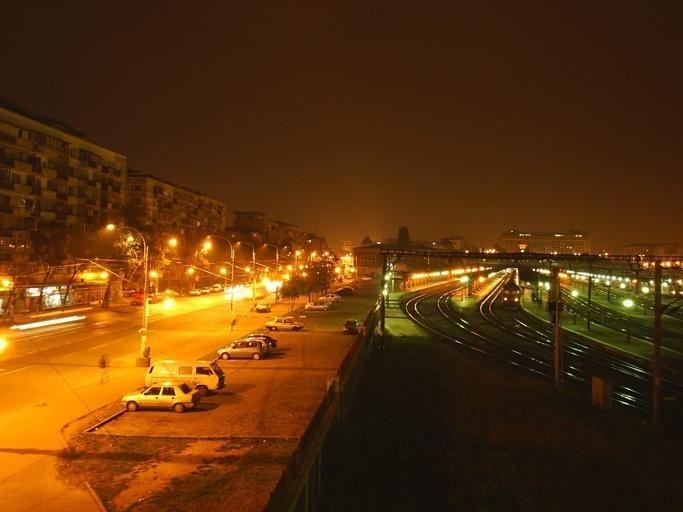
xmin=119 ymin=381 xmax=202 ymax=414
xmin=224 ymin=333 xmax=278 ymax=350
xmin=127 ymin=290 xmax=157 ymax=306
xmin=341 ymin=317 xmax=358 ymax=337
xmin=187 ymin=288 xmax=201 ymax=296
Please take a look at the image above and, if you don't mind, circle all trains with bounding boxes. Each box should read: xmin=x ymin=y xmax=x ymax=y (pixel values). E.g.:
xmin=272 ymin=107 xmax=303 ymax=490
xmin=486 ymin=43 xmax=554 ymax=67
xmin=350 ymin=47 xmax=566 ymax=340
xmin=500 ymin=267 xmax=521 ymax=313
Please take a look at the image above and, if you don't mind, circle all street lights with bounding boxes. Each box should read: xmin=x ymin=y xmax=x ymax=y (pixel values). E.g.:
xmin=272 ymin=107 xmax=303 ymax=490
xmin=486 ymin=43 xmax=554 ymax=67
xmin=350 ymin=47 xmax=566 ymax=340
xmin=379 ymin=268 xmax=392 ymax=339
xmin=105 ymin=223 xmax=153 ymax=369
xmin=531 ymin=267 xmax=683 ymax=342
xmin=459 ymin=275 xmax=469 ymax=300
xmin=201 ymin=232 xmax=356 ymax=331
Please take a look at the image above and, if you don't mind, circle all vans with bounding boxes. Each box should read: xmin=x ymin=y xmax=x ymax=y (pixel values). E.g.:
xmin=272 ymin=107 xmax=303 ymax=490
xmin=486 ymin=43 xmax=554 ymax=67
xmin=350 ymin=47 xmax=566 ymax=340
xmin=215 ymin=338 xmax=268 ymax=361
xmin=143 ymin=359 xmax=228 ymax=396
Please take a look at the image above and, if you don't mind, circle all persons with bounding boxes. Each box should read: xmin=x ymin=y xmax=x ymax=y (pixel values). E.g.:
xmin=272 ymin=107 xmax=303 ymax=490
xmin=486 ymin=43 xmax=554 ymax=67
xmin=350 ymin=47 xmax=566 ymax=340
xmin=99 ymin=355 xmax=109 ymax=383
